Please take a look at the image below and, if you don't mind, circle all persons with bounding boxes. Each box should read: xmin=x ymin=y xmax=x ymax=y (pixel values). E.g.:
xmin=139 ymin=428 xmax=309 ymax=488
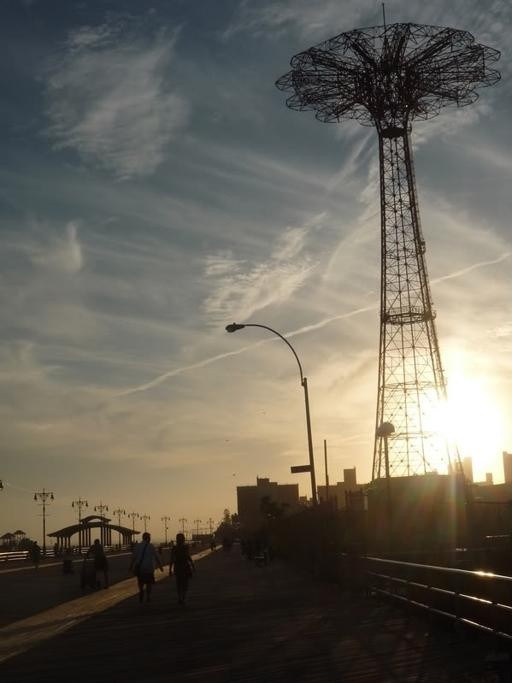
xmin=30 ymin=541 xmax=41 ymax=569
xmin=84 ymin=538 xmax=109 ymax=591
xmin=169 ymin=533 xmax=196 ymax=604
xmin=208 ymin=533 xmax=218 ymax=552
xmin=54 ymin=543 xmax=58 ymax=560
xmin=128 ymin=532 xmax=164 ymax=603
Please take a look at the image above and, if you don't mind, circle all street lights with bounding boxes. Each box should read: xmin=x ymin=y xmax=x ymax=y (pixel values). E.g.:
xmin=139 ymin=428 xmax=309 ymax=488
xmin=72 ymin=496 xmax=88 ymax=548
xmin=34 ymin=488 xmax=54 ymax=547
xmin=114 ymin=507 xmax=151 ymax=544
xmin=177 ymin=517 xmax=215 ymax=541
xmin=94 ymin=502 xmax=109 ymax=544
xmin=224 ymin=320 xmax=320 ymax=506
xmin=161 ymin=515 xmax=171 ymax=543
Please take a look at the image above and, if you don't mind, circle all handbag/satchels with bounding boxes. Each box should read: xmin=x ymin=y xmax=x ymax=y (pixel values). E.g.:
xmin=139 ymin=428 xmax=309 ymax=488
xmin=133 ymin=561 xmax=144 ymax=577
xmin=184 ymin=559 xmax=193 ymax=576
